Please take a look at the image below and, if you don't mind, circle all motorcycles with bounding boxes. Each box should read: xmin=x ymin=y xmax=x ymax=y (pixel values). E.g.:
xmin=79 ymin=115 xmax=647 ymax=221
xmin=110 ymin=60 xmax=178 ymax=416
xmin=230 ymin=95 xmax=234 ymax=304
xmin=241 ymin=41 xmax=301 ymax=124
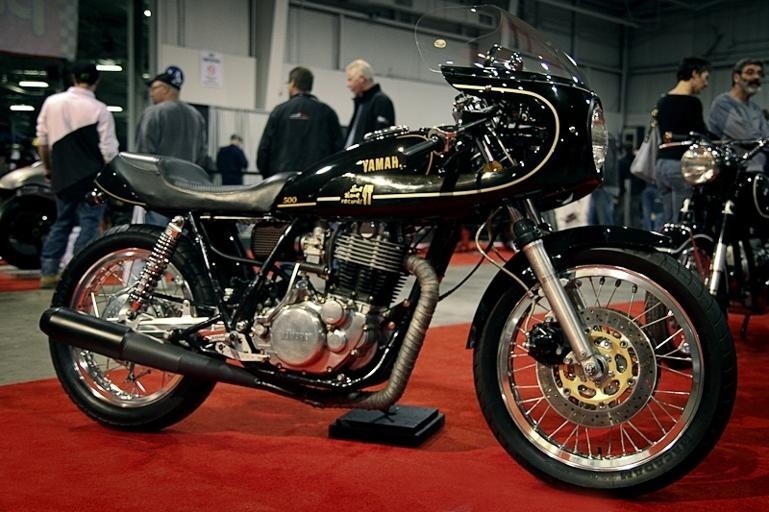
xmin=642 ymin=125 xmax=769 ymax=371
xmin=40 ymin=3 xmax=739 ymax=497
xmin=0 ymin=156 xmax=134 ymax=272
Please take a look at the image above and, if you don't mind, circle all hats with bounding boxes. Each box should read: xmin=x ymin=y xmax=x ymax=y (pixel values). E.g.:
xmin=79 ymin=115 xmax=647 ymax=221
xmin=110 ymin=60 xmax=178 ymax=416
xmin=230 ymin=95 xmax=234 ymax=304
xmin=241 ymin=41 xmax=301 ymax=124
xmin=71 ymin=59 xmax=96 ymax=85
xmin=144 ymin=74 xmax=180 ymax=90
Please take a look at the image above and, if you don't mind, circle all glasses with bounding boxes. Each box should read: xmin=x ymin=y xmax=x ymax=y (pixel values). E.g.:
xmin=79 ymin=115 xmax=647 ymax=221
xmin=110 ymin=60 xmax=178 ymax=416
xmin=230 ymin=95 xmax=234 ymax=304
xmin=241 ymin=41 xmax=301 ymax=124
xmin=738 ymin=69 xmax=765 ymax=76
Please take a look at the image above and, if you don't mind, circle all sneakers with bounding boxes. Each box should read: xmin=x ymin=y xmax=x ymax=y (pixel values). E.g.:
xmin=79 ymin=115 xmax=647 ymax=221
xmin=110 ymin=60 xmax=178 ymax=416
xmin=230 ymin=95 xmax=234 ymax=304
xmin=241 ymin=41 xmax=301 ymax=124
xmin=38 ymin=274 xmax=62 ymax=286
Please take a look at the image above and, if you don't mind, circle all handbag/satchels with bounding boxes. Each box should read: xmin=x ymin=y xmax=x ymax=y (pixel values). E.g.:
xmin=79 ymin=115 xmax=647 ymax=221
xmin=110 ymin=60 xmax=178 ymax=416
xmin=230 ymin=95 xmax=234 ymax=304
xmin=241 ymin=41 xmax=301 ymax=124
xmin=630 ymin=121 xmax=662 ymax=188
xmin=50 ymin=124 xmax=106 ymax=198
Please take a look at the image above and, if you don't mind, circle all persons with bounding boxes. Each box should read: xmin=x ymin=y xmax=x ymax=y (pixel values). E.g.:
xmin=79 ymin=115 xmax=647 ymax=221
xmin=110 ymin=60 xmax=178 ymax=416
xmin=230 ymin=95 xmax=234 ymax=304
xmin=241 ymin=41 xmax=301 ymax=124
xmin=655 ymin=56 xmax=720 ymax=222
xmin=119 ymin=73 xmax=206 ymax=305
xmin=257 ymin=66 xmax=344 ymax=180
xmin=344 ymin=59 xmax=395 ymax=148
xmin=34 ymin=62 xmax=120 ymax=288
xmin=708 ymin=58 xmax=769 ymax=142
xmin=215 ymin=134 xmax=248 ymax=184
xmin=588 ymin=134 xmax=664 ymax=231
